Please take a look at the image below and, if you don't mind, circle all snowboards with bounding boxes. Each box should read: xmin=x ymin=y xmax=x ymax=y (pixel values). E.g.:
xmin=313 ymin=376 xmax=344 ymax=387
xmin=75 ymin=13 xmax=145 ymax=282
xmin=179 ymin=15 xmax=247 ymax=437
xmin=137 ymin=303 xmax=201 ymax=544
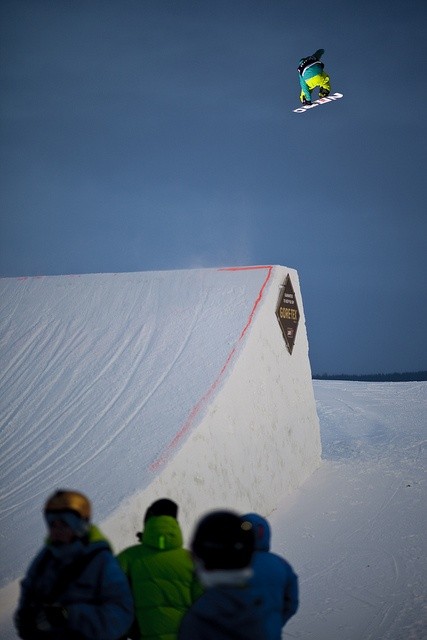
xmin=293 ymin=92 xmax=343 ymax=114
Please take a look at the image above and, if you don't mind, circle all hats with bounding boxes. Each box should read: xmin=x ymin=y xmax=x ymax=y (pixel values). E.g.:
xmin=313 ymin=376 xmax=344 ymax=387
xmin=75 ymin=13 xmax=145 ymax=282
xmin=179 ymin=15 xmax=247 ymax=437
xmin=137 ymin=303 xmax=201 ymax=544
xmin=241 ymin=514 xmax=271 ymax=549
xmin=146 ymin=499 xmax=177 ymax=519
xmin=192 ymin=513 xmax=253 ymax=571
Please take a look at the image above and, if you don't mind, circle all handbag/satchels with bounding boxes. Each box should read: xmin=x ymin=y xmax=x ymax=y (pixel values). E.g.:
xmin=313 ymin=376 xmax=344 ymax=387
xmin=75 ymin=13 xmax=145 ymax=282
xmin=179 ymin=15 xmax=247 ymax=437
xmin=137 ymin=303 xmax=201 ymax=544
xmin=18 ymin=584 xmax=72 ymax=640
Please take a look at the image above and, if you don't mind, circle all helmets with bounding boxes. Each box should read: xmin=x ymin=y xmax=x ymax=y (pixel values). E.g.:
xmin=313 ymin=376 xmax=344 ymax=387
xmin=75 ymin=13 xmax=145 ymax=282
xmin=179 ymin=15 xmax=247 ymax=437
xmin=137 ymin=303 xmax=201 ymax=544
xmin=298 ymin=58 xmax=305 ymax=67
xmin=45 ymin=492 xmax=90 ymax=522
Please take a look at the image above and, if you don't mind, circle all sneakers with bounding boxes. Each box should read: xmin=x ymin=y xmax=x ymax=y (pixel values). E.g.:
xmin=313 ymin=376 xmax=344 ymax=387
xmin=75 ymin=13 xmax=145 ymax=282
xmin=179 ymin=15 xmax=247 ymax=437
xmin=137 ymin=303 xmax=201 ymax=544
xmin=320 ymin=90 xmax=328 ymax=99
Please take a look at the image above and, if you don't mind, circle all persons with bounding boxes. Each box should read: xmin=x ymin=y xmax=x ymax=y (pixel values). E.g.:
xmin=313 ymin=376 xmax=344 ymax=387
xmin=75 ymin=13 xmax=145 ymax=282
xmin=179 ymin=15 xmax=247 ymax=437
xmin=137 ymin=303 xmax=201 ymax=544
xmin=177 ymin=510 xmax=284 ymax=639
xmin=240 ymin=513 xmax=298 ymax=630
xmin=12 ymin=492 xmax=141 ymax=638
xmin=110 ymin=500 xmax=194 ymax=640
xmin=296 ymin=49 xmax=331 ymax=105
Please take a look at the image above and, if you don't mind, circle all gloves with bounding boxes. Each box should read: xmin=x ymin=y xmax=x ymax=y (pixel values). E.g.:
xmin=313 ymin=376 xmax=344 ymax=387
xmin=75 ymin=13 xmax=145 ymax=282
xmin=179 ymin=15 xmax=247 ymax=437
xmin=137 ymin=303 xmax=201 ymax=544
xmin=306 ymin=101 xmax=311 ymax=105
